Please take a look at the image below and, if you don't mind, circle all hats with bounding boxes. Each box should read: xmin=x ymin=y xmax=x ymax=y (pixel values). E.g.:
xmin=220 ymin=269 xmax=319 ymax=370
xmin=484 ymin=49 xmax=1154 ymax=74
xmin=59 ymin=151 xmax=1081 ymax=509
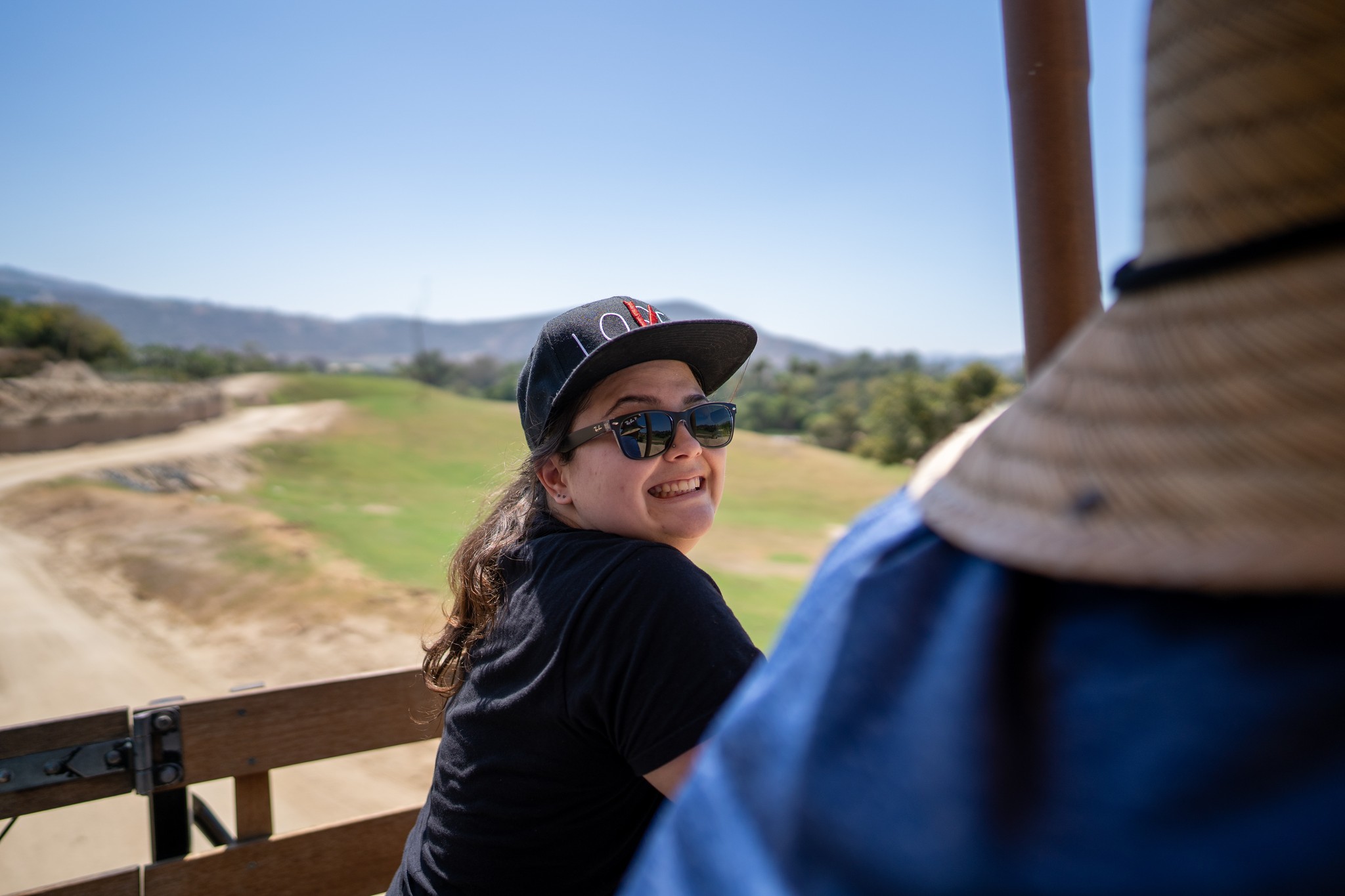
xmin=517 ymin=296 xmax=757 ymax=452
xmin=908 ymin=0 xmax=1345 ymax=600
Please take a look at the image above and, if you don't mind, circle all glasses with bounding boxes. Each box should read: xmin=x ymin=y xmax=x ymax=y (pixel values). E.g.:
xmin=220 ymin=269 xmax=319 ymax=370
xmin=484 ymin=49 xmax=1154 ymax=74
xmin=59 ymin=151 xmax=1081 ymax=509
xmin=559 ymin=402 xmax=736 ymax=461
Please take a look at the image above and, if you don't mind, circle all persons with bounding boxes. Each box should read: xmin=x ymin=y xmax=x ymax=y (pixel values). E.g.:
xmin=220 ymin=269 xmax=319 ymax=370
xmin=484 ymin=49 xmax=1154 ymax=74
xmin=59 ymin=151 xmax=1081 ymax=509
xmin=385 ymin=295 xmax=770 ymax=896
xmin=627 ymin=1 xmax=1345 ymax=896
